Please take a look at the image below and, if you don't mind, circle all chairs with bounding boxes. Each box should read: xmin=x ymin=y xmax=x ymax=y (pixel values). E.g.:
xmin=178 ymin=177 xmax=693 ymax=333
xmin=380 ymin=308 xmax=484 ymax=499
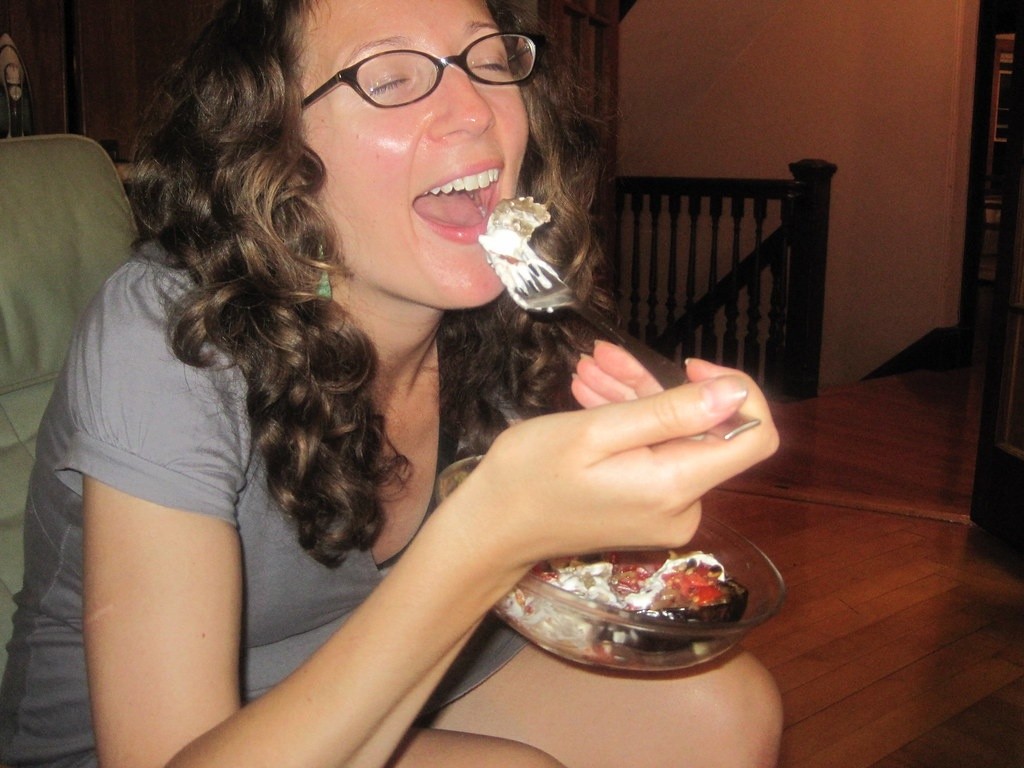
xmin=0 ymin=130 xmax=139 ymax=662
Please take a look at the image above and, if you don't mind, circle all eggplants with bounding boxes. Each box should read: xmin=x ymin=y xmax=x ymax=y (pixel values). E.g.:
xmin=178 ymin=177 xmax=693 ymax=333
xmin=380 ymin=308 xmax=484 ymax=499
xmin=594 ymin=578 xmax=748 ymax=655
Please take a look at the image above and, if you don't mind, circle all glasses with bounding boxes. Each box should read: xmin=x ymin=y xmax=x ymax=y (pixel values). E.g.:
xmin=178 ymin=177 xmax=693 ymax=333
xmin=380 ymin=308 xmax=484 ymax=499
xmin=298 ymin=30 xmax=549 ymax=109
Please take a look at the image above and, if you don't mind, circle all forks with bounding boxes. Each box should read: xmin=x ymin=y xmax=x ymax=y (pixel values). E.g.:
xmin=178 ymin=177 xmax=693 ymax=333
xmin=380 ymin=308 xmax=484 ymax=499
xmin=482 ymin=232 xmax=762 ymax=440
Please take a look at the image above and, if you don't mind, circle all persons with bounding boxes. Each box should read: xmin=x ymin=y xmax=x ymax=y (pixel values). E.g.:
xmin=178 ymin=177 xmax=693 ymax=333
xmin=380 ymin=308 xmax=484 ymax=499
xmin=3 ymin=0 xmax=786 ymax=768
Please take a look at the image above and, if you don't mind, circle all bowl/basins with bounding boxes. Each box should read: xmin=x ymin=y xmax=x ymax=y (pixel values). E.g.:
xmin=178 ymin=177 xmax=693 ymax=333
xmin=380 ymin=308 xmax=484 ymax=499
xmin=438 ymin=442 xmax=786 ymax=673
